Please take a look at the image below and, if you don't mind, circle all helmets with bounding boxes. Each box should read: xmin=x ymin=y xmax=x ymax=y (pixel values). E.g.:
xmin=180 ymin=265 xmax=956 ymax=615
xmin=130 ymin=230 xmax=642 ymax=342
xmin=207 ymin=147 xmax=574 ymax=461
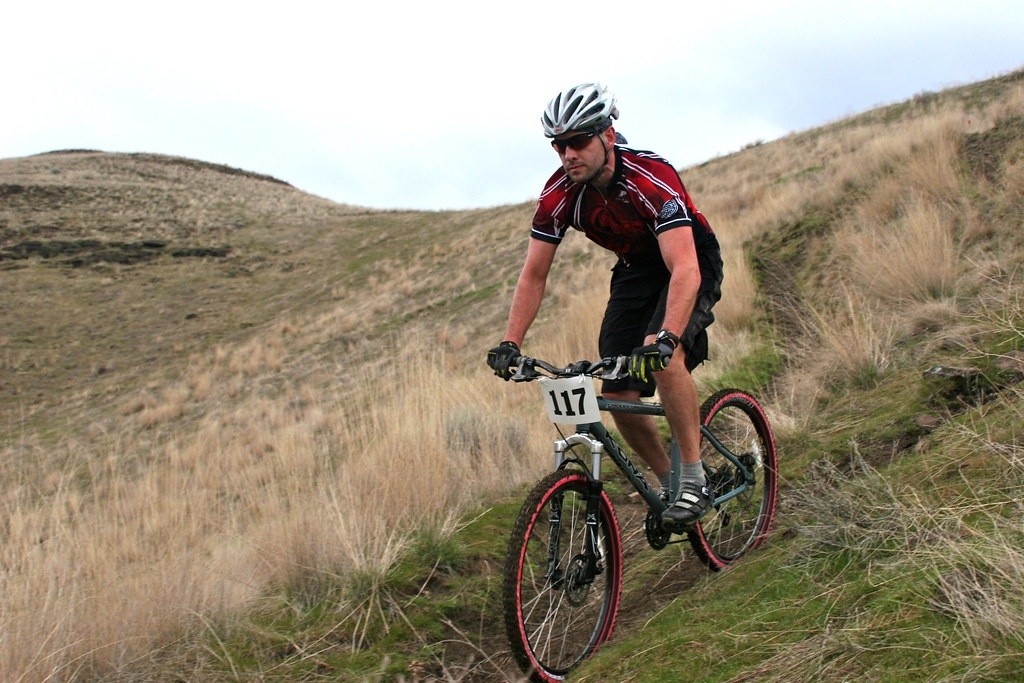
xmin=541 ymin=84 xmax=615 ymax=139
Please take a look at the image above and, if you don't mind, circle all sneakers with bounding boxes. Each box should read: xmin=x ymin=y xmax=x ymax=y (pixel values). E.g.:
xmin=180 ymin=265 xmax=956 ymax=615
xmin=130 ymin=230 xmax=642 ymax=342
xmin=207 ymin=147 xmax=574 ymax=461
xmin=659 ymin=479 xmax=717 ymax=524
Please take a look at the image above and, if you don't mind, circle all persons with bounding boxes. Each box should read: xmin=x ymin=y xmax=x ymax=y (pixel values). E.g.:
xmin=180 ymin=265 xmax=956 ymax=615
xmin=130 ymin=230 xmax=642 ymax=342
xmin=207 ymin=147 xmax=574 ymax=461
xmin=486 ymin=82 xmax=725 ymax=528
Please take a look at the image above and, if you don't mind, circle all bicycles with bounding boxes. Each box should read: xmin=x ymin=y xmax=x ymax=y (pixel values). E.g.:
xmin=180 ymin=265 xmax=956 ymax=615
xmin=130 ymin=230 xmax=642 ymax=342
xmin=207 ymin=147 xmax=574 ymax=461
xmin=503 ymin=354 xmax=778 ymax=683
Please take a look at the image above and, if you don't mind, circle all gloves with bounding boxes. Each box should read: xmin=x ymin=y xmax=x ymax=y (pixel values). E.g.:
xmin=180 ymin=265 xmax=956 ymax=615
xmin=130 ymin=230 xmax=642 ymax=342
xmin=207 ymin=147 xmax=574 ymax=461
xmin=488 ymin=341 xmax=521 ymax=382
xmin=627 ymin=332 xmax=674 ymax=384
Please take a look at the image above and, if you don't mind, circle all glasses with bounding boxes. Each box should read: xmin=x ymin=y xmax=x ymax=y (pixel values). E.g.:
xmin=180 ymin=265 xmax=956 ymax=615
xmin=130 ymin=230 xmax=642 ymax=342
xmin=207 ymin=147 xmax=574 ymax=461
xmin=550 ymin=120 xmax=612 ymax=154
